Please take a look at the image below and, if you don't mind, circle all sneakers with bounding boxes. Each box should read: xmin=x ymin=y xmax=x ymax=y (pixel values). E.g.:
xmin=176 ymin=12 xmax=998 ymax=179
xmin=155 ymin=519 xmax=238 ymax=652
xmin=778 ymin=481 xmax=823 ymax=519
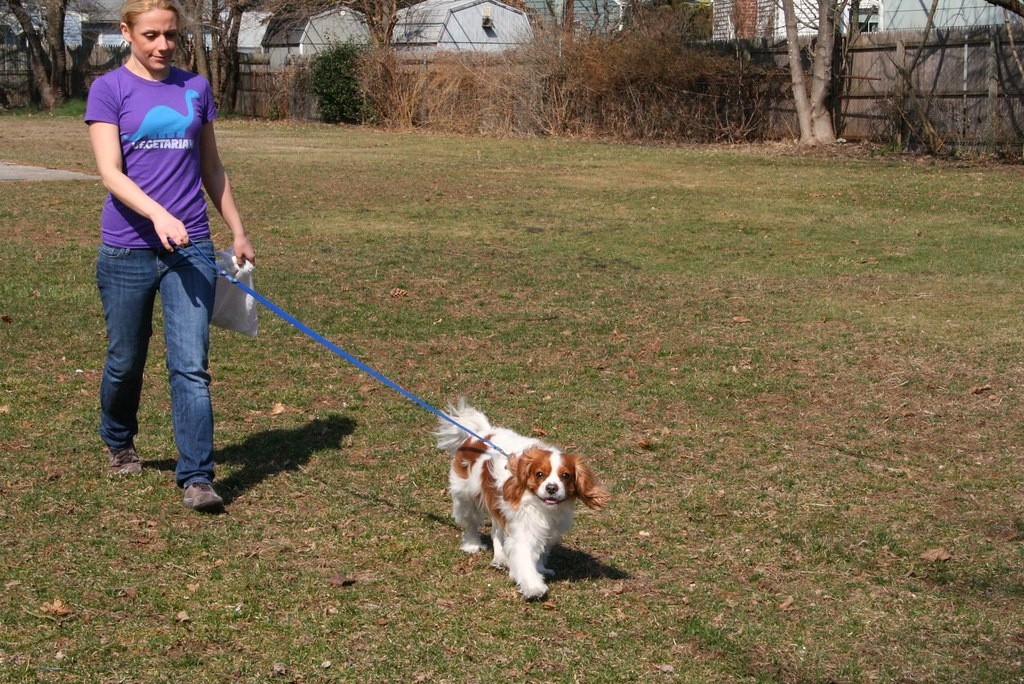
xmin=184 ymin=481 xmax=222 ymax=509
xmin=108 ymin=442 xmax=142 ymax=476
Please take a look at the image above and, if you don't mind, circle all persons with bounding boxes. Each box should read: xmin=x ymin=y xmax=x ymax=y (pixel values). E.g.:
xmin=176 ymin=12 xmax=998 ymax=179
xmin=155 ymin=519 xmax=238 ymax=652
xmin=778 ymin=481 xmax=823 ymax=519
xmin=84 ymin=0 xmax=255 ymax=512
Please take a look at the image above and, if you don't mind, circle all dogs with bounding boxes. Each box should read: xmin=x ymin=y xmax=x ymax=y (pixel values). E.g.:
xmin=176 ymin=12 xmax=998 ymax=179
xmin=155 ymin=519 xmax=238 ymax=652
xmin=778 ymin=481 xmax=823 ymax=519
xmin=433 ymin=395 xmax=613 ymax=599
